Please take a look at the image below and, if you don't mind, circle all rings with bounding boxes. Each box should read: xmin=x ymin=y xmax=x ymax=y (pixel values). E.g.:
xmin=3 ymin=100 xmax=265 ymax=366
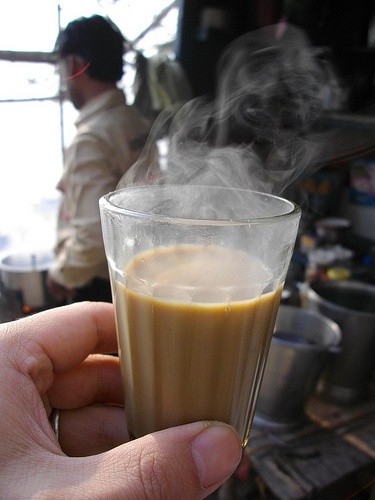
xmin=50 ymin=410 xmax=59 ymax=442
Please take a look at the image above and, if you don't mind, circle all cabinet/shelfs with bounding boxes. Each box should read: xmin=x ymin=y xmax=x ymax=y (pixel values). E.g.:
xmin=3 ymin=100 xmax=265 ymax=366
xmin=220 ymin=66 xmax=375 ymax=291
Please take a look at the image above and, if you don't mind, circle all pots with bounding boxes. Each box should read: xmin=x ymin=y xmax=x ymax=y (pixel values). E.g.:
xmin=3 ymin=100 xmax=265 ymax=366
xmin=1 ymin=254 xmax=69 ymax=306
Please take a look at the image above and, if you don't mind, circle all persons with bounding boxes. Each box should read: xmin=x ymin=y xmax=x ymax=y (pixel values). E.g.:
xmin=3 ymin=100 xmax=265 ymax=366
xmin=0 ymin=301 xmax=243 ymax=500
xmin=46 ymin=14 xmax=161 ymax=305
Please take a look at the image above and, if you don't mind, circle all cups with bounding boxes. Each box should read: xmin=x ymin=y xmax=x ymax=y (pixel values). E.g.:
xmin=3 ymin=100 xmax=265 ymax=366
xmin=254 ymin=303 xmax=343 ymax=426
xmin=98 ymin=186 xmax=300 ymax=454
xmin=300 ymin=278 xmax=375 ymax=405
